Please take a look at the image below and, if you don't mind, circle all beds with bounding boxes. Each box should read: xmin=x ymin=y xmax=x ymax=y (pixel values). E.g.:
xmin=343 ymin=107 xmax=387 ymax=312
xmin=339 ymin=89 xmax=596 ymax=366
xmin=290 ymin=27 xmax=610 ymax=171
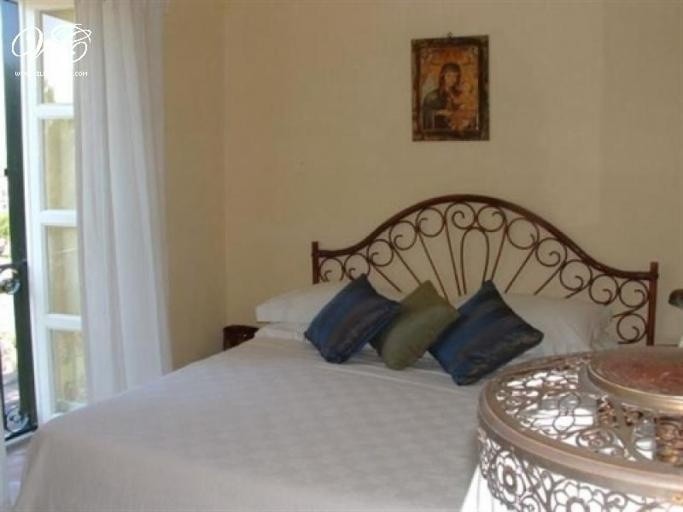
xmin=12 ymin=193 xmax=661 ymax=512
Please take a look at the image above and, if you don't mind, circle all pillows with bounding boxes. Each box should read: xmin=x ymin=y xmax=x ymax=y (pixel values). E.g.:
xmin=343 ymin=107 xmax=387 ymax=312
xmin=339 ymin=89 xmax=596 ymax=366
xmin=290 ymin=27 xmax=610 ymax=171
xmin=255 ymin=278 xmax=389 ymax=324
xmin=424 ymin=278 xmax=545 ymax=386
xmin=454 ymin=289 xmax=599 ymax=361
xmin=256 ymin=320 xmax=372 ymax=354
xmin=305 ymin=274 xmax=408 ymax=366
xmin=367 ymin=280 xmax=460 ymax=371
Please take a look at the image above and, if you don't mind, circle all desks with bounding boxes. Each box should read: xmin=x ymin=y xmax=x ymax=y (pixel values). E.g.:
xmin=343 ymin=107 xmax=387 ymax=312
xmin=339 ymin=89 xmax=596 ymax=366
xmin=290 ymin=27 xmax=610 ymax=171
xmin=474 ymin=342 xmax=683 ymax=512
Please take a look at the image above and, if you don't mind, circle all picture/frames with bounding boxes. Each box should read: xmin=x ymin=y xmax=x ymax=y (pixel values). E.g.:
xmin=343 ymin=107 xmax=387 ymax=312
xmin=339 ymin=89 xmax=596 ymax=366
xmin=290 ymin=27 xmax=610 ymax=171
xmin=411 ymin=34 xmax=490 ymax=143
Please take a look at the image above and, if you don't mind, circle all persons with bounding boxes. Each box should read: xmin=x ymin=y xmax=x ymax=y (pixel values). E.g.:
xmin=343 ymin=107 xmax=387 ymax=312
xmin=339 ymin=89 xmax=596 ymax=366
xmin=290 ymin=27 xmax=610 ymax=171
xmin=422 ymin=60 xmax=478 ymax=130
xmin=444 ymin=79 xmax=477 ymax=131
